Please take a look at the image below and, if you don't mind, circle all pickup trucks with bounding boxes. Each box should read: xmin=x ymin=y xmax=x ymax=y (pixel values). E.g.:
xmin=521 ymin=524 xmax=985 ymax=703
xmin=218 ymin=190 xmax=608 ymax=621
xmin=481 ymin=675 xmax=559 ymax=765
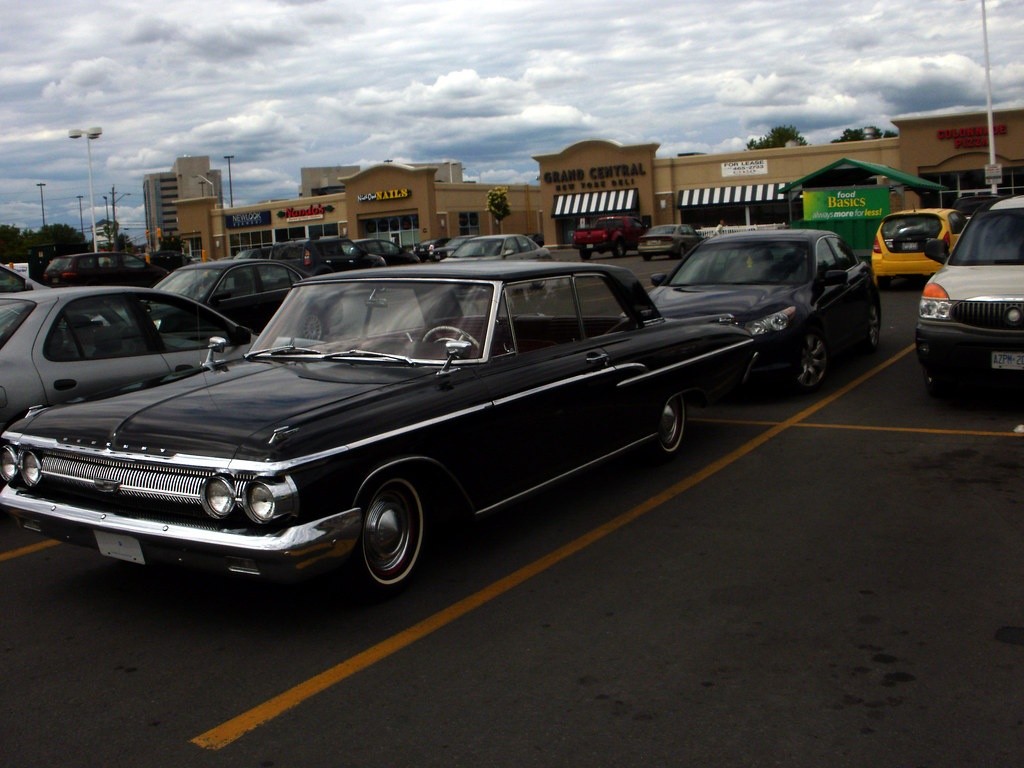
xmin=571 ymin=217 xmax=648 ymax=260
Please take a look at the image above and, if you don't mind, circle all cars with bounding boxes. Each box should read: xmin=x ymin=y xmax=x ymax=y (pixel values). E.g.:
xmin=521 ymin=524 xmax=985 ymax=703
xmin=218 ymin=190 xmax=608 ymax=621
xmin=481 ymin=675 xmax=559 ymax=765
xmin=91 ymin=259 xmax=345 ymax=342
xmin=231 ymin=236 xmax=421 ymax=276
xmin=0 ymin=263 xmax=52 ymax=292
xmin=44 ymin=252 xmax=170 ymax=288
xmin=413 ymin=235 xmax=479 ymax=262
xmin=620 ymin=195 xmax=1024 ymax=398
xmin=0 ymin=261 xmax=761 ymax=599
xmin=138 ymin=251 xmax=191 ymax=271
xmin=0 ymin=287 xmax=326 ymax=434
xmin=439 ymin=234 xmax=551 ymax=301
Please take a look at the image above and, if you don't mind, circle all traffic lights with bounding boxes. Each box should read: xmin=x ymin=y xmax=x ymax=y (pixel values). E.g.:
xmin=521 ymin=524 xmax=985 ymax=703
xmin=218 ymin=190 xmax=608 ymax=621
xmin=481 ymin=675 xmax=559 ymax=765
xmin=146 ymin=230 xmax=149 ymax=239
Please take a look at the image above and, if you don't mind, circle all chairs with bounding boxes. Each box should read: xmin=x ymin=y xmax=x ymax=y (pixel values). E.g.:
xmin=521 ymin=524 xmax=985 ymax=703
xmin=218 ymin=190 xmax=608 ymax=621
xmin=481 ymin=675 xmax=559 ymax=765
xmin=93 ymin=327 xmax=123 ymax=357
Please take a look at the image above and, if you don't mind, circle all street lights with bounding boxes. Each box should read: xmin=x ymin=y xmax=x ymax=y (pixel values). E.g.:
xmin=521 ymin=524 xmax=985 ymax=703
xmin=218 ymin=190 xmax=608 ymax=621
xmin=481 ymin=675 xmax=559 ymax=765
xmin=76 ymin=195 xmax=84 ymax=235
xmin=37 ymin=184 xmax=46 ymax=226
xmin=224 ymin=156 xmax=234 ymax=207
xmin=192 ymin=174 xmax=215 ymax=196
xmin=103 ymin=196 xmax=111 ymax=251
xmin=69 ymin=127 xmax=102 ymax=253
xmin=112 ymin=193 xmax=131 ymax=252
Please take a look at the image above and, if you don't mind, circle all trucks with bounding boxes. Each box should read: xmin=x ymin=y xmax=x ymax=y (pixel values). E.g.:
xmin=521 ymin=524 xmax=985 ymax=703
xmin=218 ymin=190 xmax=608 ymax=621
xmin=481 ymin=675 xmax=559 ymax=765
xmin=32 ymin=242 xmax=94 ymax=278
